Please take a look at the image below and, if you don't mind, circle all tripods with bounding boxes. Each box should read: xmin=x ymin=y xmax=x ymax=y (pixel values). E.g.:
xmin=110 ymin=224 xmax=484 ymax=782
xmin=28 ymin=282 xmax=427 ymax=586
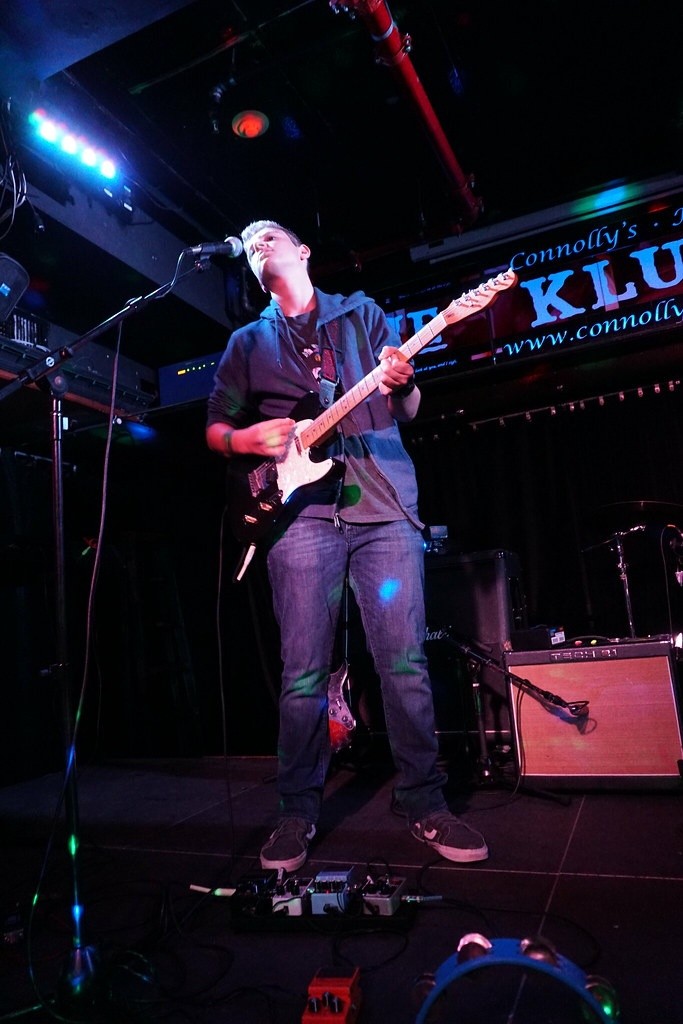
xmin=0 ymin=258 xmax=213 ymax=1024
xmin=427 ymin=637 xmax=572 ymax=809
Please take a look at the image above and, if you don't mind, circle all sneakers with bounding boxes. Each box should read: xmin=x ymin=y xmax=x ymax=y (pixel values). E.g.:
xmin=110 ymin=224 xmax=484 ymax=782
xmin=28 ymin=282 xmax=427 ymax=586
xmin=410 ymin=808 xmax=489 ymax=861
xmin=259 ymin=817 xmax=317 ymax=875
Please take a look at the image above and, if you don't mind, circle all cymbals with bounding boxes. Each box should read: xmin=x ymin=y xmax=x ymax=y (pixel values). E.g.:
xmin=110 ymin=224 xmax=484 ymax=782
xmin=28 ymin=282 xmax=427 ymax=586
xmin=590 ymin=498 xmax=683 ymax=527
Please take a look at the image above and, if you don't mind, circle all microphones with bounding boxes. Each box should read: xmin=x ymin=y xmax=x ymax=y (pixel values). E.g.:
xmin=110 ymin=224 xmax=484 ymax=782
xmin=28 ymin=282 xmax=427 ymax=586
xmin=183 ymin=236 xmax=243 ymax=258
xmin=570 ymin=704 xmax=589 ymax=715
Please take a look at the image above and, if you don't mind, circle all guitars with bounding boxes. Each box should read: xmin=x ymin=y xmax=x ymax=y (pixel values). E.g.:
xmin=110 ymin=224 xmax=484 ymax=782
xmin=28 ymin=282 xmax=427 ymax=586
xmin=223 ymin=264 xmax=524 ymax=549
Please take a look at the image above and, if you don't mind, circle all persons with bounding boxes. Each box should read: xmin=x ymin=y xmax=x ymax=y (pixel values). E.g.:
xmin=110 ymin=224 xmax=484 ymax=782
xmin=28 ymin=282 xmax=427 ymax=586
xmin=204 ymin=221 xmax=490 ymax=872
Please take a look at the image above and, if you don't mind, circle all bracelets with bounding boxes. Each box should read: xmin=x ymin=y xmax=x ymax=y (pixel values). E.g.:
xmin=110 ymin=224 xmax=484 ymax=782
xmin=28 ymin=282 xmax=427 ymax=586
xmin=224 ymin=426 xmax=235 ymax=456
xmin=388 ymin=374 xmax=415 ymax=399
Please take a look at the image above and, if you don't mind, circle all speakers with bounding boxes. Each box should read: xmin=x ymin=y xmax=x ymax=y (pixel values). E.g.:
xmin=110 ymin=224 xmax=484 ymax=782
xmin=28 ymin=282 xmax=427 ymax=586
xmin=0 ymin=253 xmax=30 ymax=323
xmin=503 ymin=639 xmax=683 ymax=792
xmin=423 ymin=546 xmax=529 ymax=762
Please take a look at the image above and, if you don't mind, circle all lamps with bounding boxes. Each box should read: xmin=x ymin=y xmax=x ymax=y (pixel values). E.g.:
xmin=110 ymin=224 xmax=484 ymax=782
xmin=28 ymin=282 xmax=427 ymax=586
xmin=231 ymin=109 xmax=269 ymax=139
